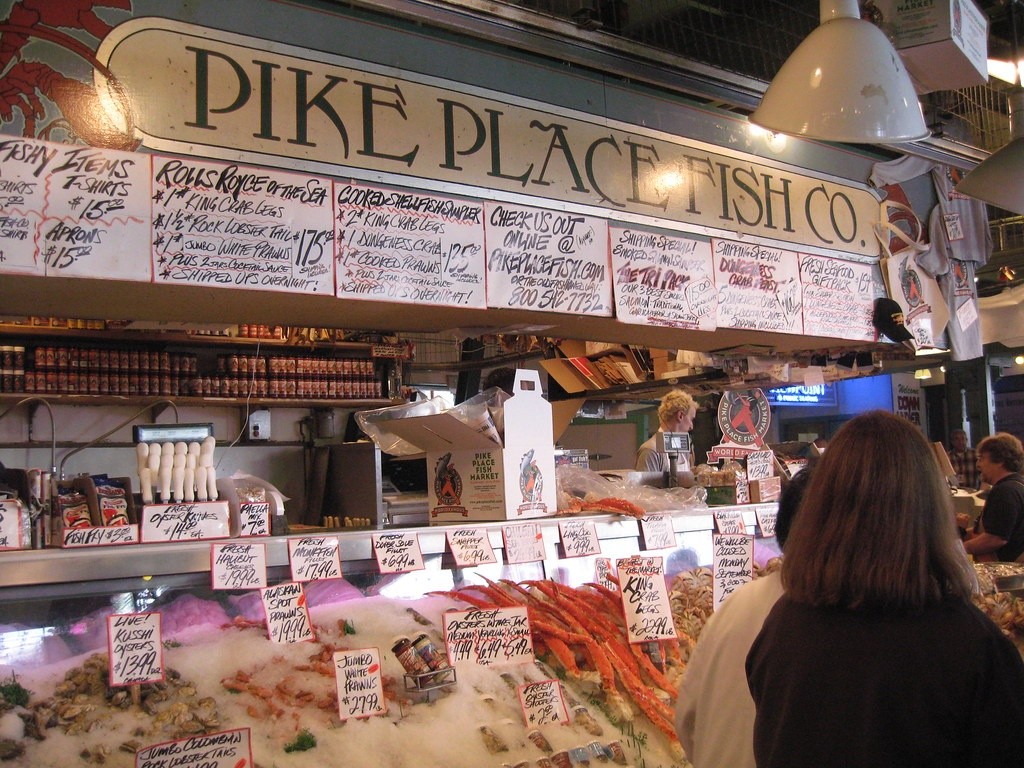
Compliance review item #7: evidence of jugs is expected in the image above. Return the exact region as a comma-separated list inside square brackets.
[299, 406, 334, 439]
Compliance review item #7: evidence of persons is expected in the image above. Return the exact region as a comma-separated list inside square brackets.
[949, 428, 987, 490]
[483, 366, 528, 408]
[675, 467, 812, 768]
[635, 390, 699, 487]
[961, 432, 1024, 562]
[745, 410, 1024, 768]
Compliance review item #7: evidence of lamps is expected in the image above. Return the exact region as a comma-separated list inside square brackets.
[61, 400, 213, 481]
[954, 135, 1024, 218]
[749, 0, 931, 143]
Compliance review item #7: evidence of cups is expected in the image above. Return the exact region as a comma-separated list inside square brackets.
[871, 351, 883, 374]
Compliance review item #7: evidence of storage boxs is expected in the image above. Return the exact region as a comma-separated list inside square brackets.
[762, 441, 820, 487]
[375, 367, 557, 524]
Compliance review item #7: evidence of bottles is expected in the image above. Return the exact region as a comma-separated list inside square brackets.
[1, 314, 381, 399]
[722, 458, 734, 470]
[392, 635, 451, 687]
[386, 370, 402, 400]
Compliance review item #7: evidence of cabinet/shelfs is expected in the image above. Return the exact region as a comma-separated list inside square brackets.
[0, 323, 401, 451]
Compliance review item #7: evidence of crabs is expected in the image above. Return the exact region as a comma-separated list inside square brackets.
[662, 557, 784, 645]
[424, 571, 687, 744]
